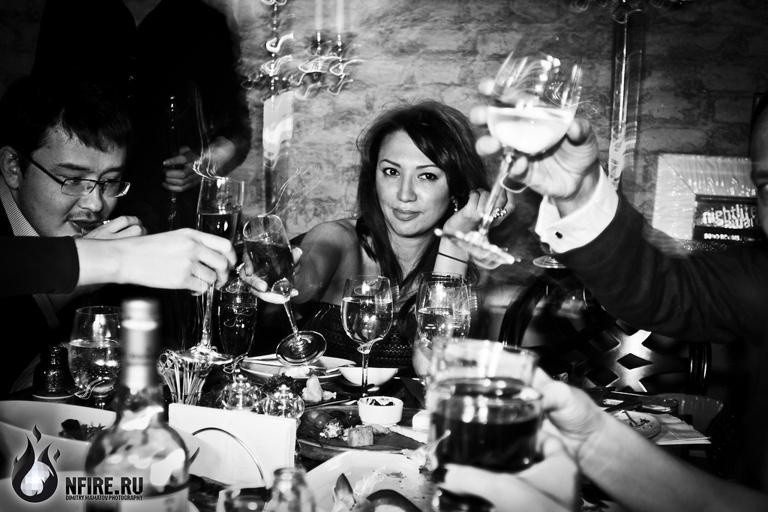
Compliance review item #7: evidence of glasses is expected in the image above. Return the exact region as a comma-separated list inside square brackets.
[26, 151, 131, 199]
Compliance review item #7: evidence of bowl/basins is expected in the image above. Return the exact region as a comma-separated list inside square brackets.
[411, 335, 517, 386]
[358, 395, 404, 426]
[0, 399, 119, 476]
[646, 393, 722, 434]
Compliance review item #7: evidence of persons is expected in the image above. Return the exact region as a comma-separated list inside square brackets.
[238, 97, 520, 384]
[0, 223, 242, 302]
[0, 85, 188, 401]
[463, 72, 768, 497]
[0, 0, 253, 339]
[401, 331, 767, 510]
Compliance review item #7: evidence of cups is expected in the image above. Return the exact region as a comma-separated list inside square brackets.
[420, 273, 542, 512]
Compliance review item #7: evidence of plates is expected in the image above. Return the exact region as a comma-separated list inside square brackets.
[241, 352, 357, 382]
[295, 405, 428, 462]
[341, 366, 399, 387]
[598, 406, 660, 440]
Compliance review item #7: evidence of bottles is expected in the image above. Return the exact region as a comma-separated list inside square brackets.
[85, 300, 188, 512]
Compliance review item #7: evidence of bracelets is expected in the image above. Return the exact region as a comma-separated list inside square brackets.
[435, 250, 468, 265]
[428, 272, 466, 290]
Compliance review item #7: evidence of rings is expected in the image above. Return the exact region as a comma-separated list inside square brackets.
[492, 207, 506, 219]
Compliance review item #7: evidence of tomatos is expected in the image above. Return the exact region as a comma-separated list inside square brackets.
[315, 415, 327, 428]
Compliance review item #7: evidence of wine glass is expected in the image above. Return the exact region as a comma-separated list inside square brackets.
[337, 273, 395, 402]
[181, 177, 327, 410]
[434, 45, 580, 267]
[67, 307, 126, 414]
